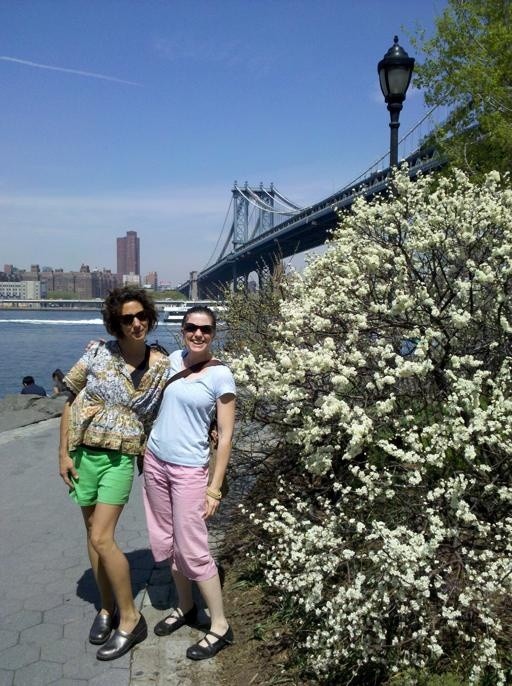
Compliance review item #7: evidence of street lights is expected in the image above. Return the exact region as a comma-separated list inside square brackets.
[377, 34, 416, 203]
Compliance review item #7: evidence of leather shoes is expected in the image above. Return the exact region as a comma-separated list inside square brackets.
[96, 611, 148, 660]
[185, 624, 233, 660]
[89, 605, 117, 645]
[154, 604, 197, 636]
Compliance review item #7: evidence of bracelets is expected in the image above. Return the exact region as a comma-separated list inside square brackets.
[207, 489, 222, 500]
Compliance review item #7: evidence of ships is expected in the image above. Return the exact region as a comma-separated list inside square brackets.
[162, 295, 231, 323]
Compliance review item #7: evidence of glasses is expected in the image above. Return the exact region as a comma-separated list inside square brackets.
[183, 323, 215, 334]
[121, 311, 148, 326]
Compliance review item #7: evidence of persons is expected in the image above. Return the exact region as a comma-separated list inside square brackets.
[21, 376, 46, 397]
[50, 368, 69, 396]
[59, 286, 167, 661]
[139, 305, 236, 660]
